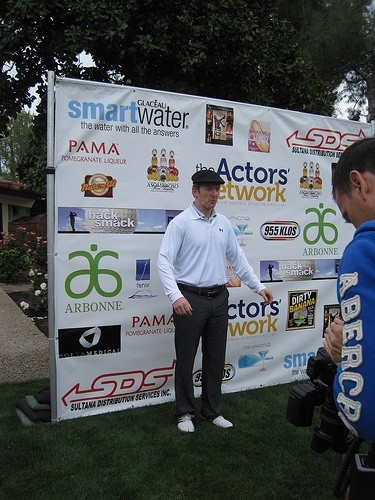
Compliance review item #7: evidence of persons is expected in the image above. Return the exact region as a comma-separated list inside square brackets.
[322, 134, 375, 500]
[158, 169, 274, 432]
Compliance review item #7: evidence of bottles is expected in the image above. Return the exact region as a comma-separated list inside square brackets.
[147, 149, 159, 175]
[168, 150, 179, 176]
[299, 162, 308, 183]
[314, 163, 322, 184]
[158, 148, 169, 175]
[308, 162, 315, 183]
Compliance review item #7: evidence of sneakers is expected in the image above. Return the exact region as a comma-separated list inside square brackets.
[209, 415, 233, 428]
[178, 413, 195, 432]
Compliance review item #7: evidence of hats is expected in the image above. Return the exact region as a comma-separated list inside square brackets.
[192, 170, 225, 186]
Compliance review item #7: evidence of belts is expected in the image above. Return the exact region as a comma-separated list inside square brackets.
[178, 284, 225, 299]
